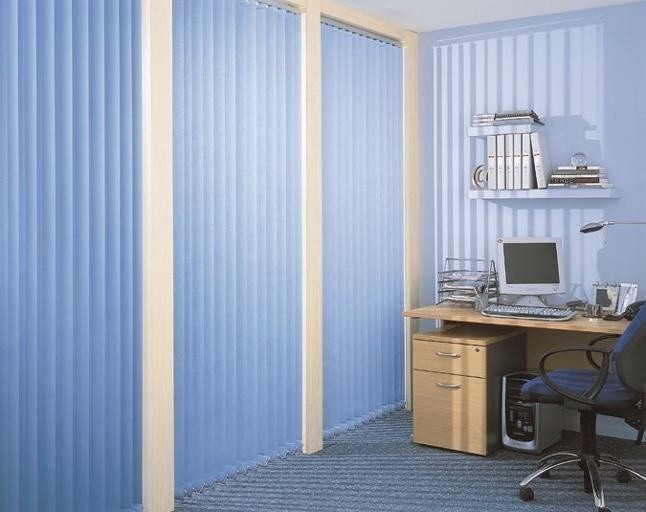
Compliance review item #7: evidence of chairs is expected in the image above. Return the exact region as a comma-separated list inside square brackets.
[520, 306, 645, 511]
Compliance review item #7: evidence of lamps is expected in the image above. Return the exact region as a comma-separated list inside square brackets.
[580, 219, 645, 233]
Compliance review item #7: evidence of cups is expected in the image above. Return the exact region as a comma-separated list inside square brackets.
[475, 294, 489, 311]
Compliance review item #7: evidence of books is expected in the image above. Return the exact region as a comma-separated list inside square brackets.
[486, 132, 613, 189]
[444, 269, 496, 305]
[472, 110, 547, 126]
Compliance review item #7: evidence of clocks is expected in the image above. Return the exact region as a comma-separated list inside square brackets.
[570, 153, 586, 167]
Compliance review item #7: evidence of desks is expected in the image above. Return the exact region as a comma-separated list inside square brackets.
[402, 294, 635, 457]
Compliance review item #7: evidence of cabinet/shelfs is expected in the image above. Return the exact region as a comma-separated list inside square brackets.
[468, 125, 619, 198]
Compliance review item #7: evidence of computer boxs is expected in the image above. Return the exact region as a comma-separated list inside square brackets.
[502, 371, 562, 454]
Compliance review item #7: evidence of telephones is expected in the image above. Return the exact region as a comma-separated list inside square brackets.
[626, 301, 646, 321]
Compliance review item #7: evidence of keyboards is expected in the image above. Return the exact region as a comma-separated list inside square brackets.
[480, 303, 576, 321]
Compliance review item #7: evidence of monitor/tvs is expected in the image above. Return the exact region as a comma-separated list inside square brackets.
[496, 237, 567, 308]
